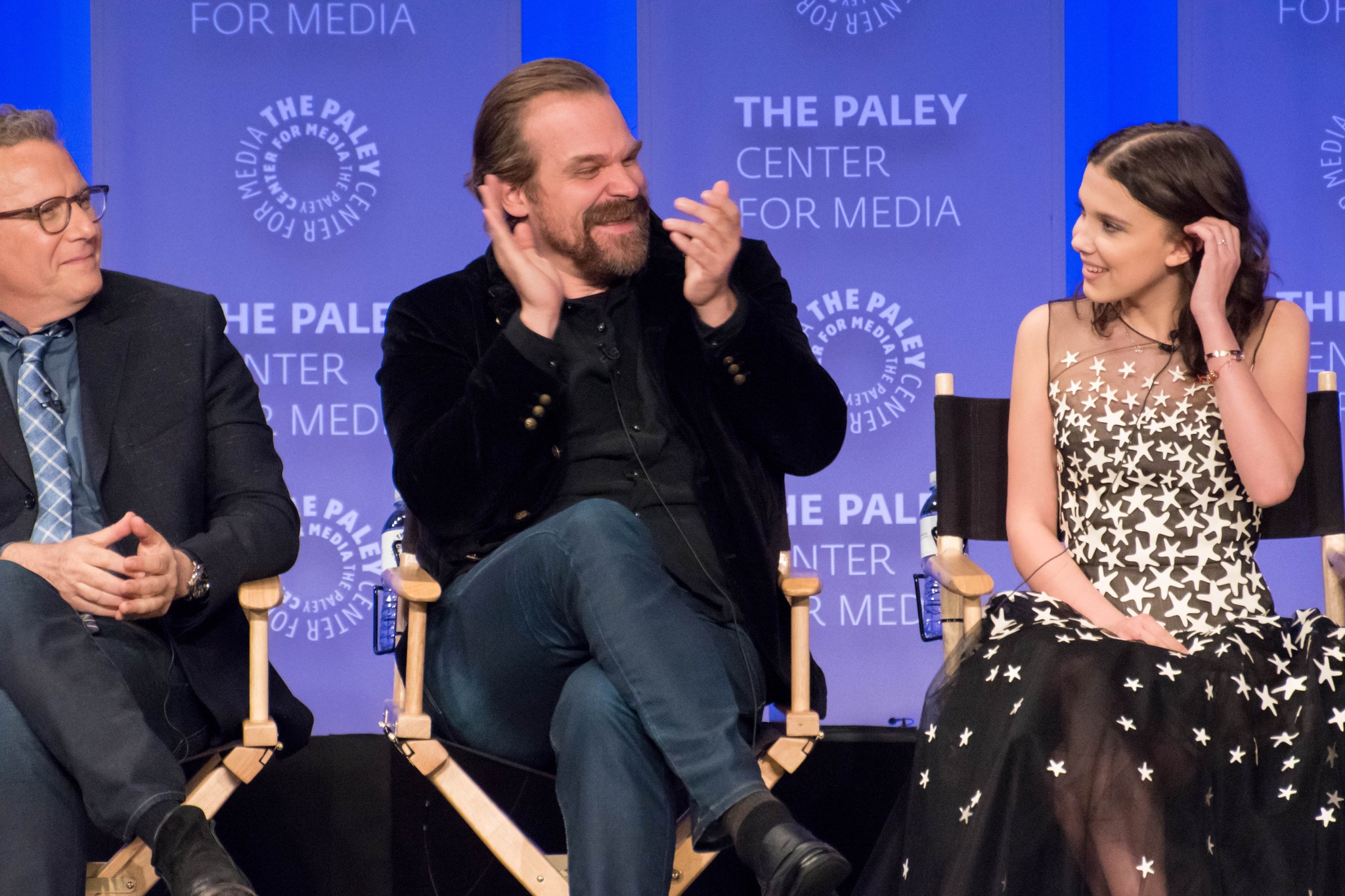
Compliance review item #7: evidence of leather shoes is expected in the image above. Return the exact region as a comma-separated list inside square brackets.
[754, 823, 851, 896]
[152, 804, 254, 896]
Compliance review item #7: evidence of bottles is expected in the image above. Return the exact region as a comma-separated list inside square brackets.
[379, 489, 408, 652]
[918, 471, 942, 636]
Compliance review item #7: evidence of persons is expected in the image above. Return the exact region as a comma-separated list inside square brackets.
[0, 103, 301, 896]
[373, 55, 855, 896]
[887, 123, 1344, 895]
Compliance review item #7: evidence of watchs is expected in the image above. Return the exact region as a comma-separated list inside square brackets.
[178, 545, 211, 601]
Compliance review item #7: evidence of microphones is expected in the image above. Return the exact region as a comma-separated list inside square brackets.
[41, 390, 64, 413]
[598, 343, 622, 359]
[1171, 330, 1184, 342]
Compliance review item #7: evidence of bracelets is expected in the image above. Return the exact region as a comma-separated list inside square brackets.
[1205, 354, 1246, 385]
[1204, 350, 1243, 358]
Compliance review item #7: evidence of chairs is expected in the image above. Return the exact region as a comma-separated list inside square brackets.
[86, 575, 285, 896]
[381, 503, 825, 896]
[924, 371, 1345, 677]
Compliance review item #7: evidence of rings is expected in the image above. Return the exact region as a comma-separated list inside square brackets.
[1217, 240, 1228, 247]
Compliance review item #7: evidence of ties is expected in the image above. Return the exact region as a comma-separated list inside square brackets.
[0, 320, 71, 543]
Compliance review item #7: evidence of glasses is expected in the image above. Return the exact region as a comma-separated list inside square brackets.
[0, 184, 110, 234]
